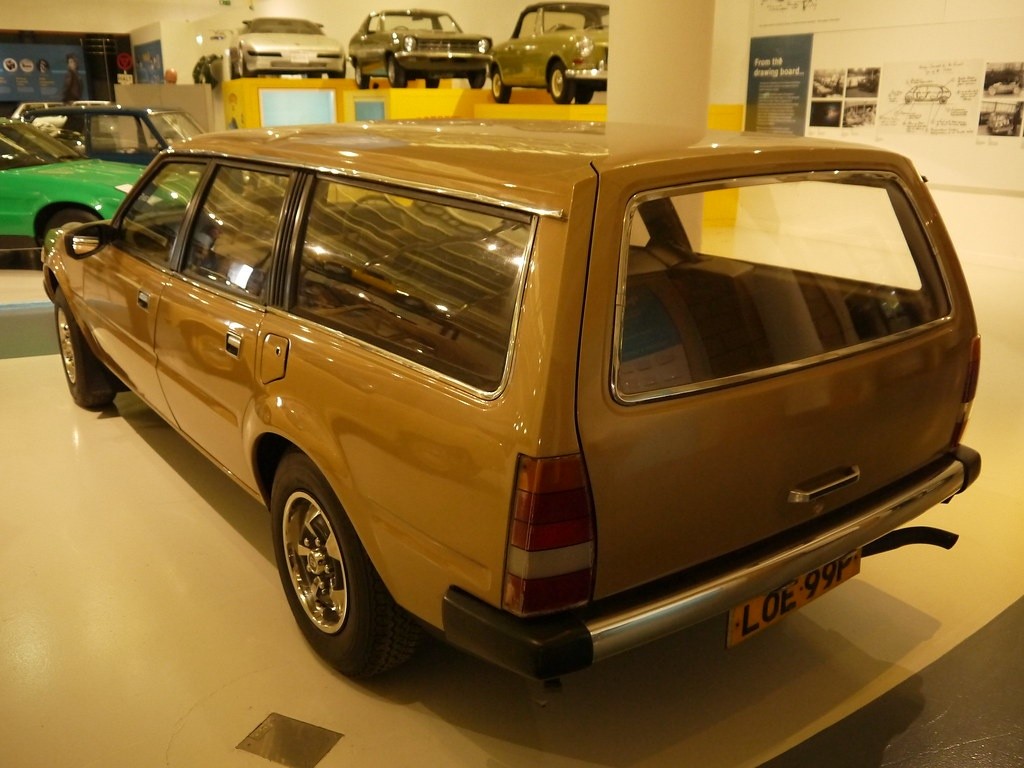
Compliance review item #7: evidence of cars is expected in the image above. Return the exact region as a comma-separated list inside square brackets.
[237, 16, 349, 79]
[43, 115, 983, 678]
[490, 5, 610, 104]
[349, 9, 494, 91]
[17, 106, 216, 172]
[1, 114, 200, 259]
